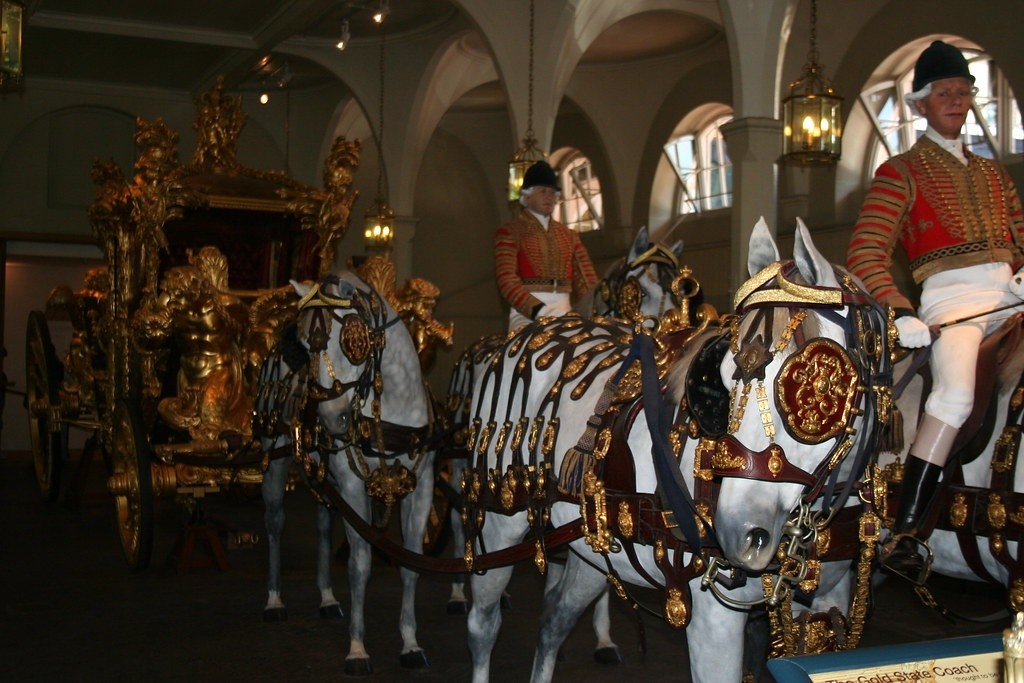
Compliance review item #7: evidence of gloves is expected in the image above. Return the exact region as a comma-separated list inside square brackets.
[535, 305, 565, 320]
[894, 316, 932, 349]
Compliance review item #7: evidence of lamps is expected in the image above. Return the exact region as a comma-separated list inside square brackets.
[507, 0, 547, 204]
[363, 8, 394, 254]
[336, 20, 351, 51]
[277, 72, 287, 87]
[0, 0, 23, 88]
[779, 0, 843, 166]
[370, 0, 391, 23]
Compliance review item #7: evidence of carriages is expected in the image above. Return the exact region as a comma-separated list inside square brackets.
[21, 75, 458, 679]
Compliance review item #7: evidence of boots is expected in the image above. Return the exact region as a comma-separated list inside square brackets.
[882, 453, 944, 576]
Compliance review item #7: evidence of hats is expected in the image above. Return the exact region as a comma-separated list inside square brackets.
[521, 160, 562, 190]
[912, 40, 975, 92]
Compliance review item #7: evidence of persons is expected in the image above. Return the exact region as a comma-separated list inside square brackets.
[496, 160, 600, 335]
[846, 41, 1024, 569]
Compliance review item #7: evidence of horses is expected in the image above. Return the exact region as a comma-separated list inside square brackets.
[440, 217, 1024, 683]
[251, 273, 430, 675]
